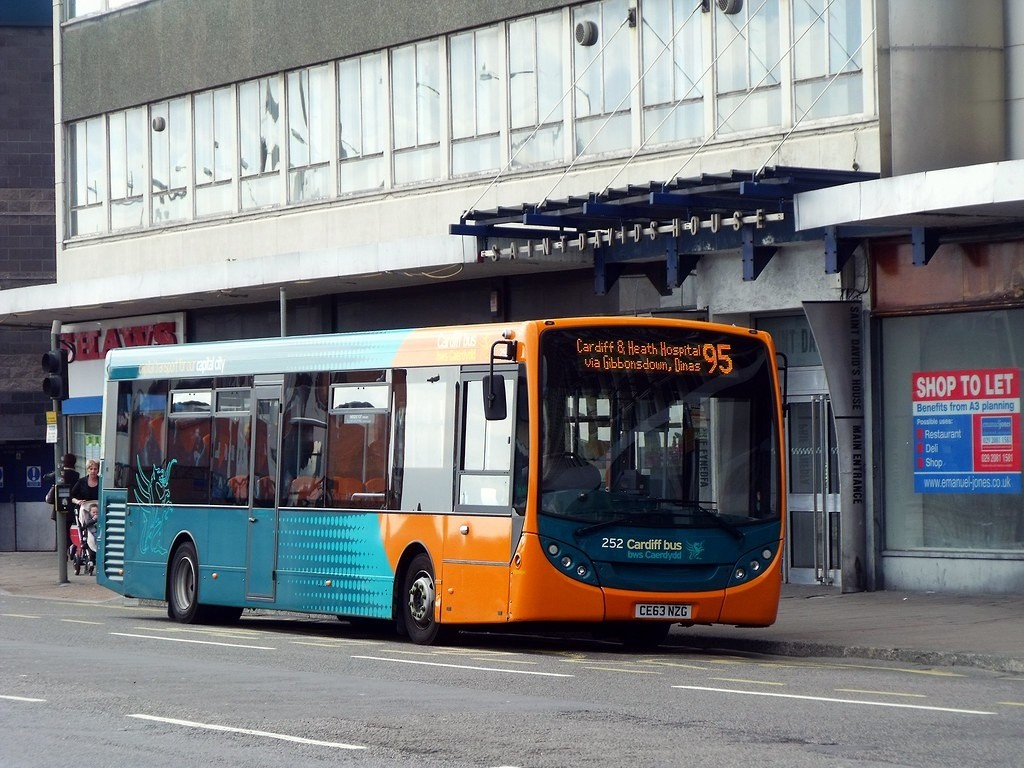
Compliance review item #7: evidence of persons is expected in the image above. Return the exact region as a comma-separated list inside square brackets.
[44, 454, 78, 562]
[70, 459, 100, 575]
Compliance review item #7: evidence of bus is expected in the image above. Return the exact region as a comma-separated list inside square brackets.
[91, 314, 795, 643]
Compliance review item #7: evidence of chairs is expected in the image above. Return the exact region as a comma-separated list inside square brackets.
[212, 470, 385, 508]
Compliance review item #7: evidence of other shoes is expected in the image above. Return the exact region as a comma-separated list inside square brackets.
[84, 570, 89, 574]
[69, 544, 77, 561]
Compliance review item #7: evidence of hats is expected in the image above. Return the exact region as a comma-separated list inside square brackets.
[63, 454, 77, 469]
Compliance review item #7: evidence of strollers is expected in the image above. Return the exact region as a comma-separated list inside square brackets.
[70, 498, 100, 576]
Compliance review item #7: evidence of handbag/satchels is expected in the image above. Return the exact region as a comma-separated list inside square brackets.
[45, 483, 55, 504]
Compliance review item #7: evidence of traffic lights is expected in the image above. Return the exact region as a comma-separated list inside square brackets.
[40, 347, 71, 401]
